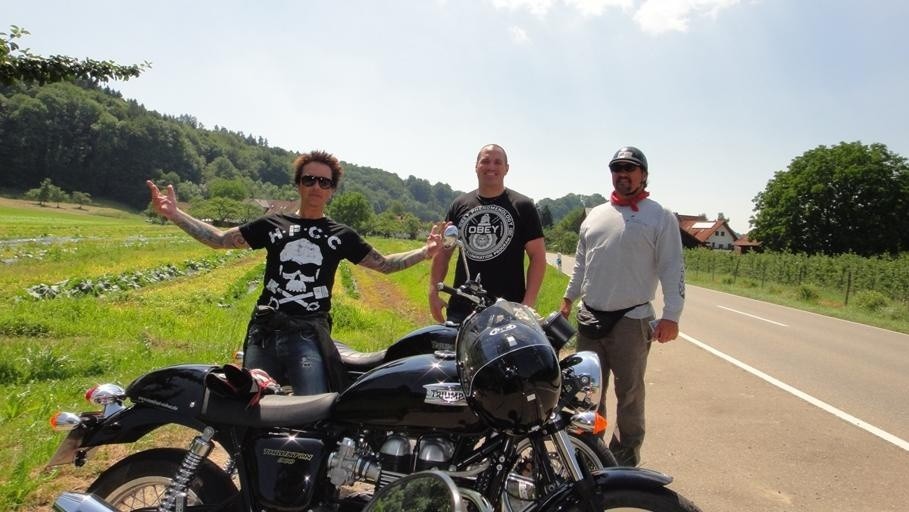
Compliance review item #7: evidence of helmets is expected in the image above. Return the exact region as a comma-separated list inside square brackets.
[609, 146, 648, 171]
[455, 301, 561, 436]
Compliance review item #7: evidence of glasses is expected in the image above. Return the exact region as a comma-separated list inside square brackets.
[611, 163, 637, 173]
[300, 174, 333, 190]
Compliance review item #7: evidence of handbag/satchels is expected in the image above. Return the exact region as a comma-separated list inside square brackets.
[574, 303, 634, 339]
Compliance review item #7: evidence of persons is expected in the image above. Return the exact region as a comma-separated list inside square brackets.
[427, 142, 549, 330]
[143, 149, 455, 398]
[559, 146, 688, 469]
